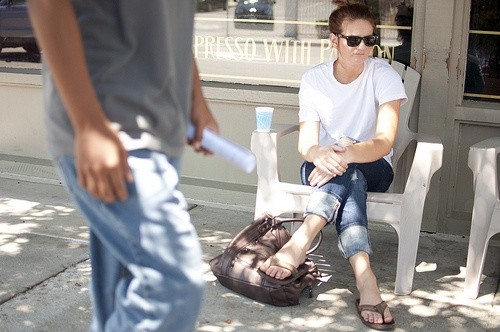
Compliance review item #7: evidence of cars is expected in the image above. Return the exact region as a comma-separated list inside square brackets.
[234, 0, 276, 31]
[0, 0, 41, 62]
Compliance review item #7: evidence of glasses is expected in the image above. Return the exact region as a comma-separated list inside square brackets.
[335, 33, 379, 47]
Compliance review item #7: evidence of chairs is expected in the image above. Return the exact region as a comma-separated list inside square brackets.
[460, 136, 500, 301]
[250, 57, 444, 296]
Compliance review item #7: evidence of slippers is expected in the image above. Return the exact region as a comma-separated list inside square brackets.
[356, 298, 396, 329]
[257, 254, 309, 284]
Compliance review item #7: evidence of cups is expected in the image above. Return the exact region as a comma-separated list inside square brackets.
[256, 107, 274, 133]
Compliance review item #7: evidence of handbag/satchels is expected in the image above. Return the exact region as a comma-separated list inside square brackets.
[209, 211, 323, 306]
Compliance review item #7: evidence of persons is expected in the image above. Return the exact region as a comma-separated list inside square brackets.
[26, 0, 218, 332]
[257, 0, 408, 332]
[382, 2, 414, 69]
